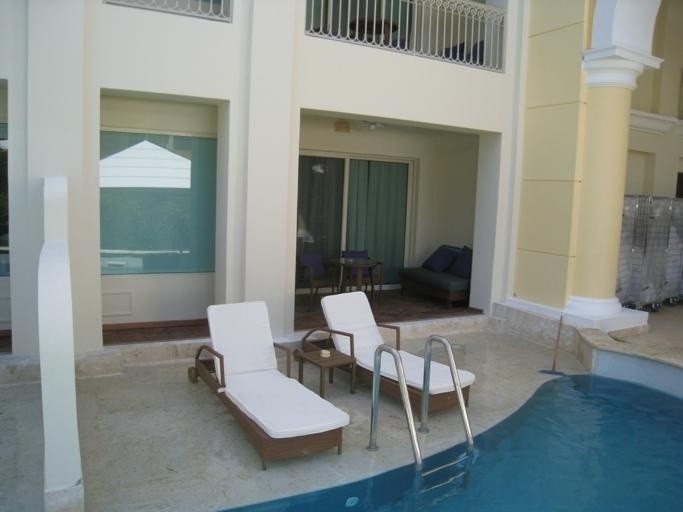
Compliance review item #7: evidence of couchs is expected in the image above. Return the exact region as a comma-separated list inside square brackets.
[399, 245, 473, 310]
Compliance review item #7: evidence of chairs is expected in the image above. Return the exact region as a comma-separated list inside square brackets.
[342, 250, 384, 292]
[321, 290, 476, 422]
[188, 299, 349, 470]
[303, 245, 338, 312]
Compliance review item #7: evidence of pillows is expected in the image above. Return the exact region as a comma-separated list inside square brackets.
[308, 246, 326, 279]
[451, 245, 472, 279]
[423, 246, 459, 273]
[346, 251, 369, 276]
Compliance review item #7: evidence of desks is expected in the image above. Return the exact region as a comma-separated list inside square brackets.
[298, 348, 357, 397]
[330, 257, 376, 302]
[350, 18, 398, 47]
